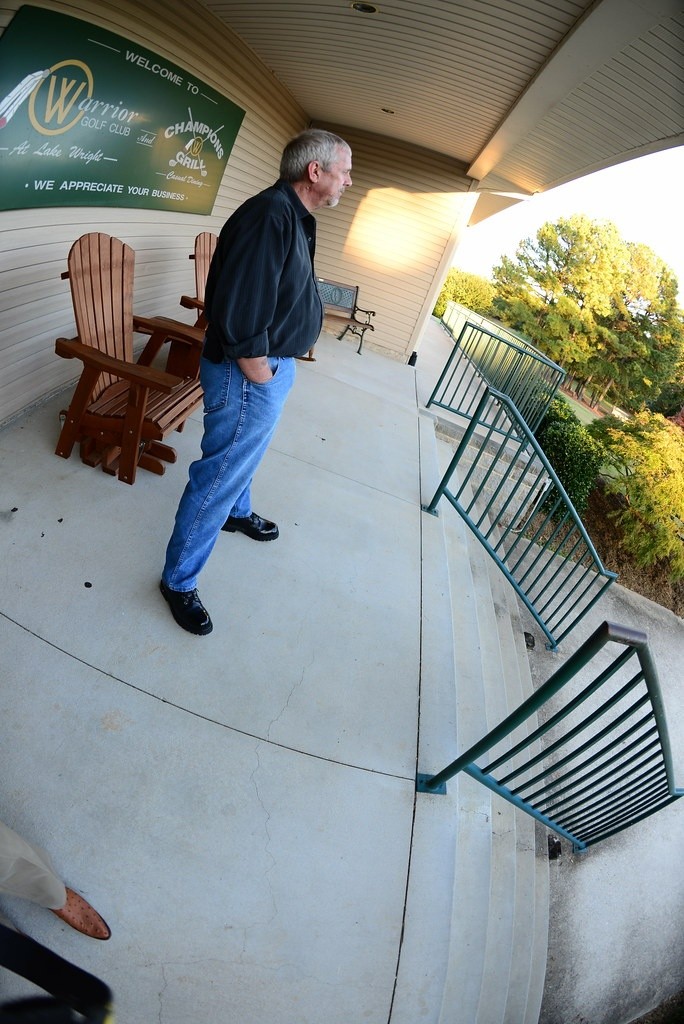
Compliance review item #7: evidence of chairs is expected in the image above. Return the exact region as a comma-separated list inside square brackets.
[180, 232, 317, 362]
[48, 232, 206, 486]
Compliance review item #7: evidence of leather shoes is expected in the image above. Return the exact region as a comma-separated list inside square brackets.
[46, 885, 111, 940]
[219, 511, 279, 542]
[158, 577, 214, 636]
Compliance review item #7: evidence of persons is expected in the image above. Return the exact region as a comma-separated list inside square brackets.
[158, 129, 354, 638]
[0, 820, 113, 941]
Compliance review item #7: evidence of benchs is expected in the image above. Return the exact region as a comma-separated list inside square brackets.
[315, 276, 376, 356]
